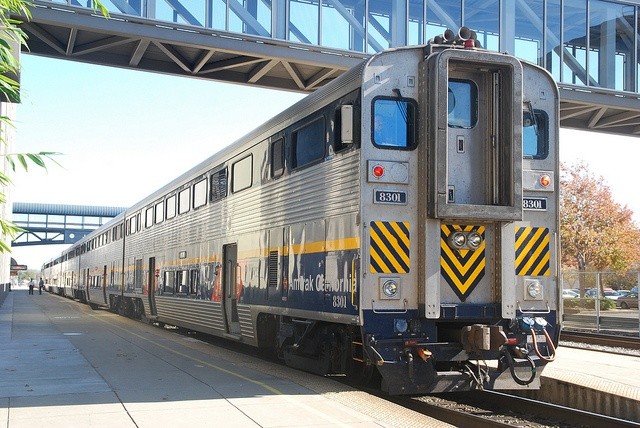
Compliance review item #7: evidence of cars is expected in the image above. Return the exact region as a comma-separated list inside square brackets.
[563, 283, 640, 309]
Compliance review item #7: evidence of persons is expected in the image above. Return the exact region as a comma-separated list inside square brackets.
[38, 277, 44, 295]
[374, 114, 385, 145]
[27, 277, 34, 294]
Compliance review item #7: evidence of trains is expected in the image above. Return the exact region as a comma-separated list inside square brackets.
[41, 25, 563, 403]
